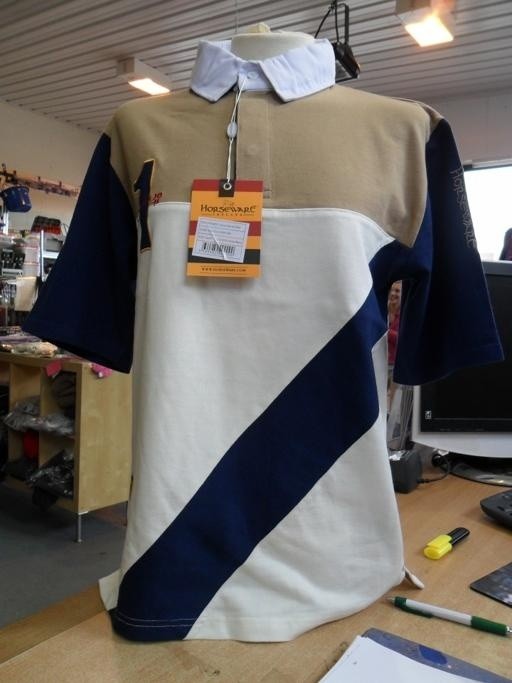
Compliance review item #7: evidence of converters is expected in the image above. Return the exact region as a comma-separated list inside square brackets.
[387, 449, 423, 493]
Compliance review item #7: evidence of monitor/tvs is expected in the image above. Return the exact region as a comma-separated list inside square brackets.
[410, 259, 512, 487]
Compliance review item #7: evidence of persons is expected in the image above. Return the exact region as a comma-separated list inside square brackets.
[19, 28, 506, 646]
[387, 279, 402, 419]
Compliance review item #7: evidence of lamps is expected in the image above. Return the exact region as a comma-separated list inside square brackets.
[396, 0, 456, 49]
[116, 57, 171, 95]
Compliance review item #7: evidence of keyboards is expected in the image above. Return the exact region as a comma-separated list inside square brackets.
[479, 489, 512, 531]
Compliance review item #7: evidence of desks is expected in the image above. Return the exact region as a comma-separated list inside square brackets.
[1, 470, 511, 683]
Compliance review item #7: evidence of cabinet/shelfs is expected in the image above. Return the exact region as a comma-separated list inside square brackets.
[0, 349, 133, 544]
[0, 229, 66, 282]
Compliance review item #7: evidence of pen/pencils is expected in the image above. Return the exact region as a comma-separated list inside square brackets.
[385, 595, 512, 635]
